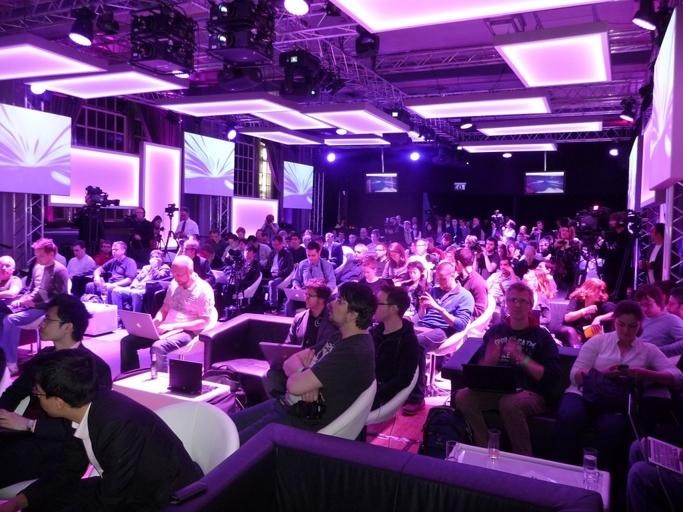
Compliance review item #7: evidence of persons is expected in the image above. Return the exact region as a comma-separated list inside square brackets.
[1, 207, 683, 511]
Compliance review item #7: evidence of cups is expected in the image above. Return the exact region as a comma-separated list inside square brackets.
[487, 430, 500, 460]
[446, 440, 457, 462]
[583, 447, 598, 480]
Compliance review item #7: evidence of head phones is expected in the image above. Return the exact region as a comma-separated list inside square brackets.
[617, 217, 626, 225]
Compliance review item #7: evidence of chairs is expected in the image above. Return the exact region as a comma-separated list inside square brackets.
[0, 245, 672, 512]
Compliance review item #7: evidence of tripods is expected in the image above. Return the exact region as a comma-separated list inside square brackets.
[219, 272, 246, 316]
[164, 231, 179, 252]
[614, 236, 651, 301]
[565, 244, 601, 300]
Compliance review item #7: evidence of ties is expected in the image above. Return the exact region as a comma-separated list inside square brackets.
[181, 222, 185, 231]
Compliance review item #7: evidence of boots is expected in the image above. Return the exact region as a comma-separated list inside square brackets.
[219, 308, 231, 321]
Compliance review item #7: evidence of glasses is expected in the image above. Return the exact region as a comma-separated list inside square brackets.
[42, 317, 62, 322]
[510, 296, 529, 306]
[304, 292, 319, 297]
[31, 387, 47, 397]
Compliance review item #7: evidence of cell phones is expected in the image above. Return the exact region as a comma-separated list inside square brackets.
[168, 482, 208, 505]
[618, 364, 628, 371]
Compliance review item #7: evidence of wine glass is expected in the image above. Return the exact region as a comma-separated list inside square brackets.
[101, 285, 108, 307]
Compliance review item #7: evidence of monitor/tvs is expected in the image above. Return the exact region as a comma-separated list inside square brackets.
[363, 171, 399, 193]
[523, 171, 568, 196]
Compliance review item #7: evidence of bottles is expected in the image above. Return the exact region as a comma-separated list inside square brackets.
[150, 353, 157, 379]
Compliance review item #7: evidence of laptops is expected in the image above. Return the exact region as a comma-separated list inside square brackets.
[119, 310, 166, 340]
[168, 359, 218, 397]
[628, 393, 683, 475]
[461, 364, 524, 394]
[284, 288, 307, 301]
[259, 342, 304, 370]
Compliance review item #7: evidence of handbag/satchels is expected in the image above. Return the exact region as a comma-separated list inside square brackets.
[462, 362, 517, 394]
[582, 367, 636, 411]
[285, 390, 326, 420]
[267, 365, 286, 397]
[417, 405, 466, 458]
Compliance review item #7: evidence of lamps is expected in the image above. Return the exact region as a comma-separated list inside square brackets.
[619, 100, 637, 122]
[68, 7, 93, 47]
[459, 117, 473, 130]
[225, 122, 238, 140]
[631, 0, 657, 31]
